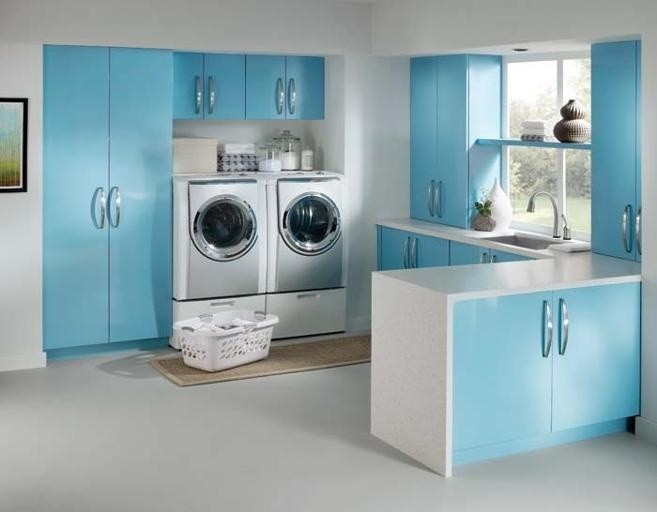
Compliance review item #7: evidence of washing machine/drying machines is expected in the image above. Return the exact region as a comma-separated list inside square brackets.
[172, 175, 267, 301]
[267, 170, 351, 294]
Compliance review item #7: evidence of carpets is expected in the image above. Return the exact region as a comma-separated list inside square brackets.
[145, 332, 370, 387]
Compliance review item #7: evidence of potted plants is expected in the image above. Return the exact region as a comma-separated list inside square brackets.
[472, 200, 496, 232]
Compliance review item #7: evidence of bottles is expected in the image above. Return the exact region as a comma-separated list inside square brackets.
[258, 143, 282, 172]
[301, 145, 314, 171]
[273, 129, 302, 171]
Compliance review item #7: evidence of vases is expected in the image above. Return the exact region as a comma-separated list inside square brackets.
[487, 177, 513, 230]
[554, 97, 591, 142]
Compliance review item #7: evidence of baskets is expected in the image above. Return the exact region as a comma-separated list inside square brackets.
[173, 309, 280, 373]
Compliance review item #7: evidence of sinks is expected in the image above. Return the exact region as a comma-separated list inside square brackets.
[482, 236, 565, 251]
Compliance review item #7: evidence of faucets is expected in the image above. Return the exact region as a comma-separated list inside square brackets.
[526, 191, 561, 237]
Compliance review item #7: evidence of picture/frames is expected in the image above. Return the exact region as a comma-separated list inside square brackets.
[0, 96, 29, 193]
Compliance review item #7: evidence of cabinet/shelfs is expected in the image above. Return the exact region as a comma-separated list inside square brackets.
[450, 239, 537, 266]
[452, 279, 640, 467]
[409, 53, 501, 229]
[42, 44, 173, 350]
[175, 50, 244, 121]
[590, 40, 641, 262]
[376, 225, 449, 271]
[243, 53, 325, 120]
